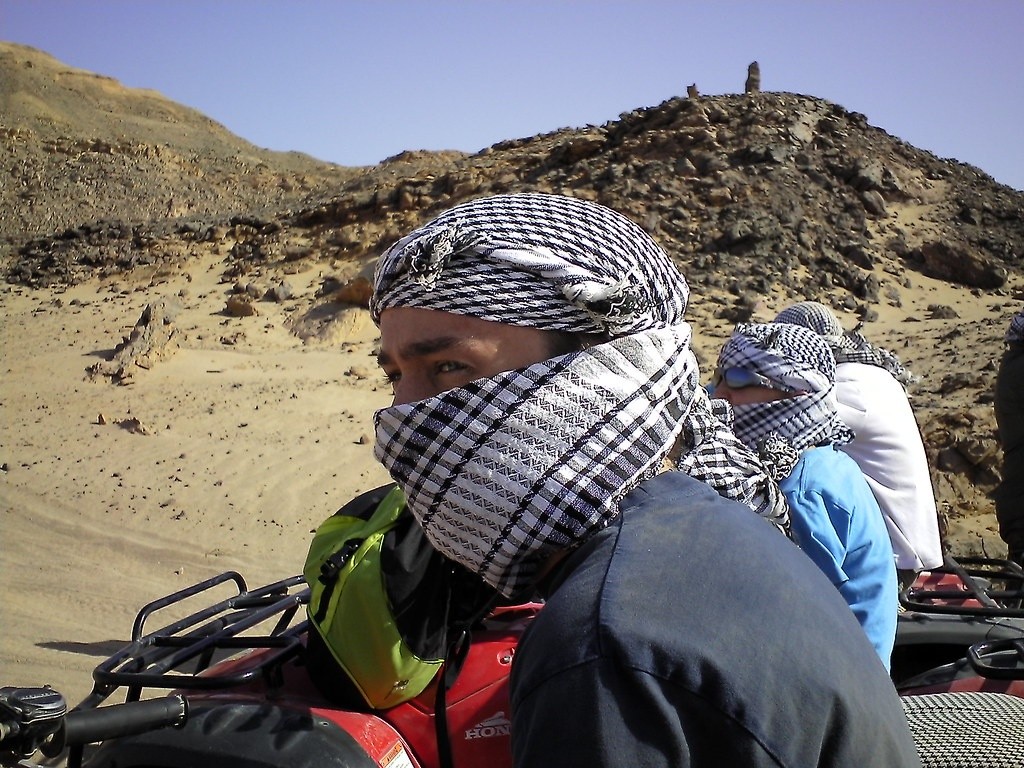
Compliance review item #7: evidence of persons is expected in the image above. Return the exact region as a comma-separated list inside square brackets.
[993, 309, 1024, 609]
[774, 301, 943, 589]
[710, 322, 898, 678]
[369, 193, 924, 768]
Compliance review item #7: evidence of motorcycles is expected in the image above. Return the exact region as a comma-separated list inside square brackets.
[1, 551, 1024, 768]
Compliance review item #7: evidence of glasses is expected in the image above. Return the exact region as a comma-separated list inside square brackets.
[713, 367, 790, 393]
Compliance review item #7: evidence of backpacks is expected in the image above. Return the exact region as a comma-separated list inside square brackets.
[302, 480, 496, 768]
